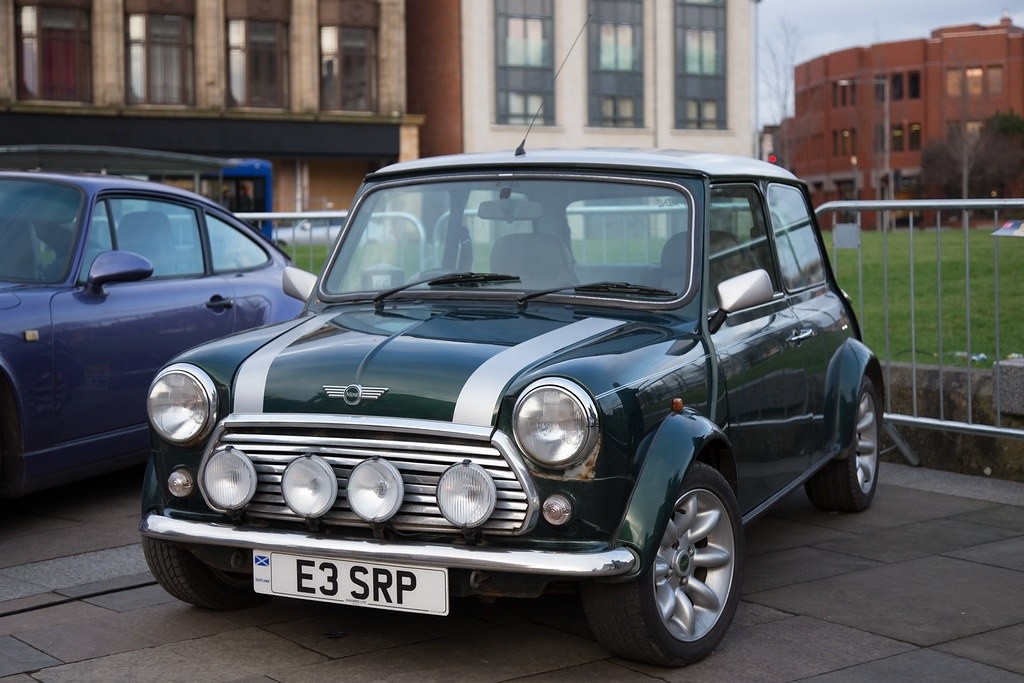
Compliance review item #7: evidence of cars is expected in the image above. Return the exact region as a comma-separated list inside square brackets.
[0, 169, 309, 498]
[134, 142, 887, 670]
[272, 217, 385, 250]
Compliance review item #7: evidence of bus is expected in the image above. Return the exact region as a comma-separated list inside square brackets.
[0, 143, 276, 246]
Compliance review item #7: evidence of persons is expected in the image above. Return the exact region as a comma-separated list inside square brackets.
[31, 188, 106, 285]
[219, 182, 250, 213]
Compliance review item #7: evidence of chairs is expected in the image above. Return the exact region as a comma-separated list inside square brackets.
[661, 232, 759, 306]
[117, 211, 177, 275]
[489, 233, 574, 292]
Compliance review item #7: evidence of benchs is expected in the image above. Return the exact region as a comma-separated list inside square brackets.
[575, 264, 660, 287]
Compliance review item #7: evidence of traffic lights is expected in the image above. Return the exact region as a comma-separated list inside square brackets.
[768, 152, 777, 165]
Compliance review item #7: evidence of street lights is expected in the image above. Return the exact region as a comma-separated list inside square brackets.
[838, 75, 891, 231]
